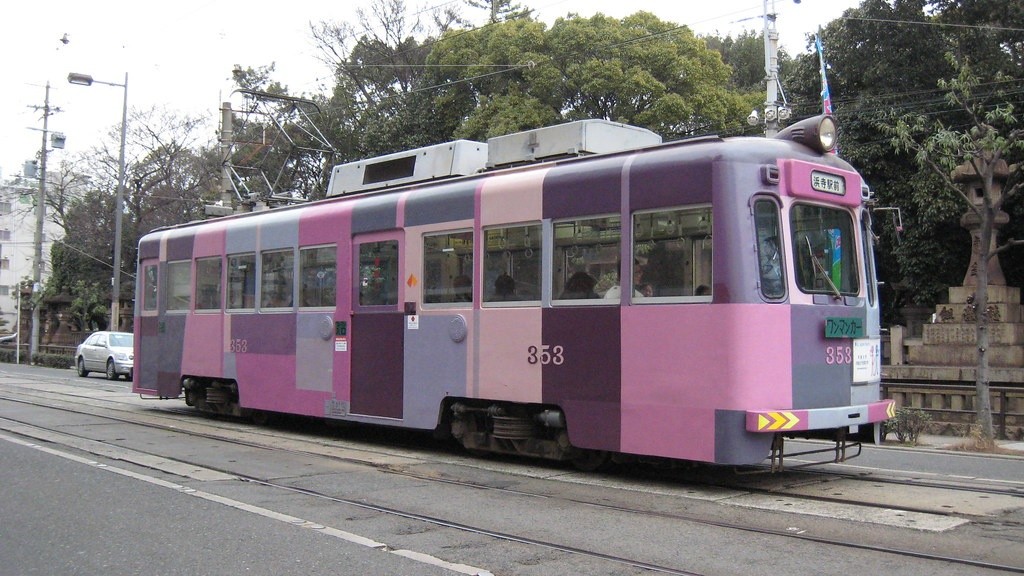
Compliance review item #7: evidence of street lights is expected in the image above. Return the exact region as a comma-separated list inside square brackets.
[67, 72, 129, 331]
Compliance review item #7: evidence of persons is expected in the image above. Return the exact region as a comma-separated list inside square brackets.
[195, 284, 218, 309]
[638, 282, 657, 297]
[289, 283, 310, 307]
[487, 276, 522, 302]
[695, 284, 712, 295]
[603, 258, 644, 299]
[446, 275, 472, 302]
[763, 236, 805, 287]
[559, 271, 600, 300]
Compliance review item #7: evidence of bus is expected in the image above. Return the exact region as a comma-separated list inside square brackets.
[130, 111, 904, 469]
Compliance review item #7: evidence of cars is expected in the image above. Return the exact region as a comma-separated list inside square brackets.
[74, 330, 134, 380]
[0, 332, 18, 344]
